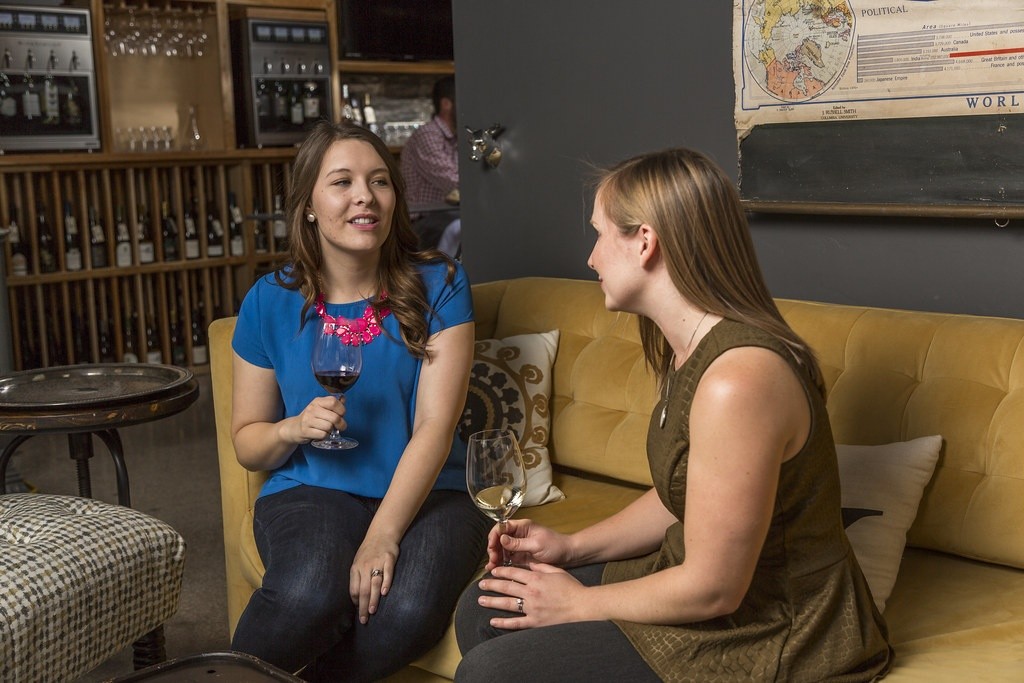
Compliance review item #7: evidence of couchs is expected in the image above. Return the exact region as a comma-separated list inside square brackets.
[208, 277, 1024, 683]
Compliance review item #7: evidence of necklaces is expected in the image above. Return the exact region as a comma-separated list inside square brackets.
[660, 311, 708, 428]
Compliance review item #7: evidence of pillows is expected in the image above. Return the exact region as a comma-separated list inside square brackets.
[836, 435, 943, 614]
[455, 328, 566, 510]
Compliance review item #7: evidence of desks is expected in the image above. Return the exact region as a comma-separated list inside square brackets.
[1, 363, 201, 508]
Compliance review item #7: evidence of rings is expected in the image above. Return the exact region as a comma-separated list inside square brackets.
[517, 598, 523, 613]
[371, 570, 383, 577]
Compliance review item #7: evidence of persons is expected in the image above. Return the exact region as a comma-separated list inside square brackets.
[454, 149, 897, 683]
[402, 76, 462, 256]
[230, 125, 489, 683]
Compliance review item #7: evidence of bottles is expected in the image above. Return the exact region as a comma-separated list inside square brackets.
[98, 313, 208, 369]
[112, 104, 203, 155]
[8, 195, 288, 277]
[340, 84, 378, 135]
[0, 71, 85, 134]
[256, 79, 320, 133]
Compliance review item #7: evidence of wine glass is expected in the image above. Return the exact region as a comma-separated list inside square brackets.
[464, 428, 529, 585]
[103, 3, 210, 60]
[310, 321, 363, 448]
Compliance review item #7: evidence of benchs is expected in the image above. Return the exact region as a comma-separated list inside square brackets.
[0, 491, 187, 683]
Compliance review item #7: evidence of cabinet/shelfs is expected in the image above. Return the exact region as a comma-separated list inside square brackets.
[0, 144, 413, 374]
